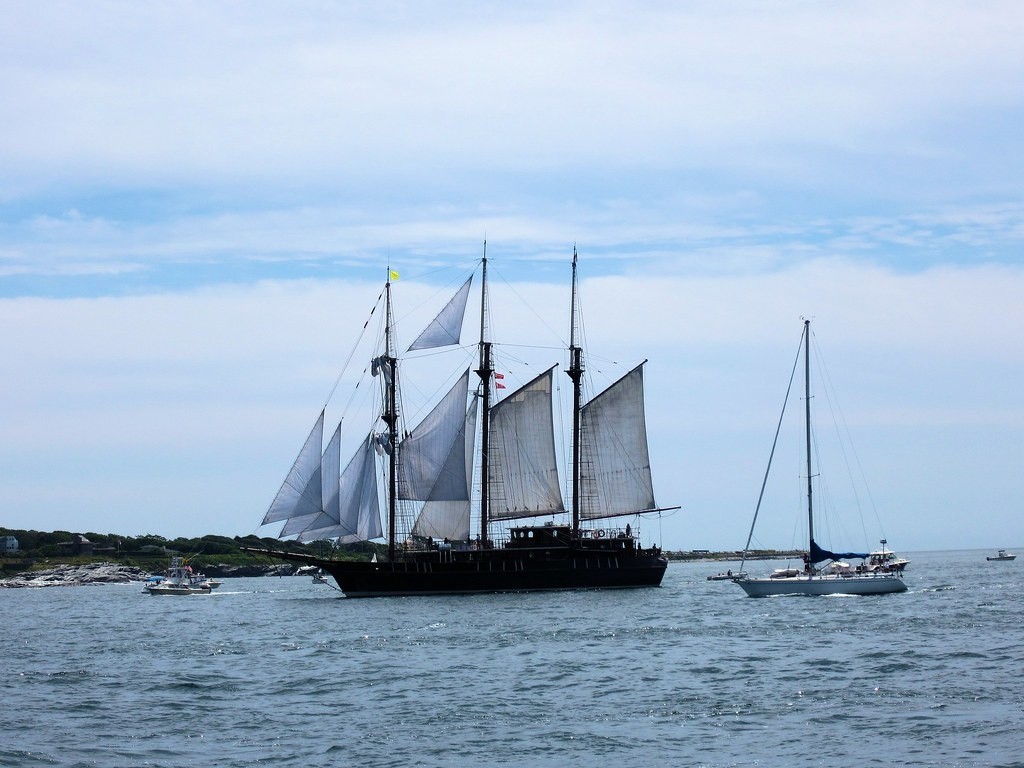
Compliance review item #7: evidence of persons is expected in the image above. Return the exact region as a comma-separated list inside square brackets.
[476, 534, 480, 550]
[626, 524, 630, 538]
[157, 580, 161, 585]
[728, 568, 732, 576]
[444, 537, 448, 544]
[467, 535, 470, 540]
[885, 558, 889, 577]
[426, 536, 432, 551]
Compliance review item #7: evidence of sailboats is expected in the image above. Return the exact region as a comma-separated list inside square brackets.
[730, 314, 908, 600]
[236, 227, 683, 599]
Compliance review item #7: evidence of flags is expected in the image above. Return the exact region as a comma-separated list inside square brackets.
[390, 270, 398, 280]
[495, 372, 504, 380]
[496, 382, 506, 388]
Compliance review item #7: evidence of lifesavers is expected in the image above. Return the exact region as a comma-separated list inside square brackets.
[593, 531, 599, 539]
[598, 530, 605, 537]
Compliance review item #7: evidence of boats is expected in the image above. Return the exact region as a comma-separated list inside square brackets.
[310, 573, 330, 585]
[855, 538, 910, 574]
[706, 557, 746, 581]
[987, 550, 1017, 562]
[140, 550, 225, 595]
[767, 565, 801, 577]
